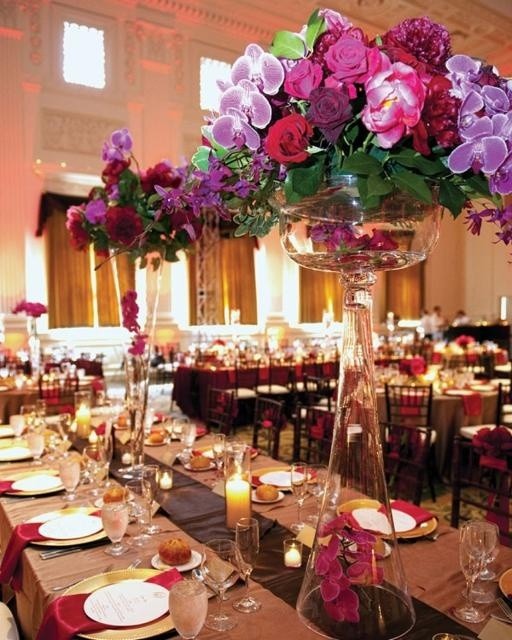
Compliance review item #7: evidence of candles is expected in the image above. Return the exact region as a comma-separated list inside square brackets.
[121, 452, 132, 464]
[90, 431, 98, 443]
[284, 544, 300, 565]
[160, 472, 172, 489]
[78, 407, 91, 438]
[225, 472, 251, 530]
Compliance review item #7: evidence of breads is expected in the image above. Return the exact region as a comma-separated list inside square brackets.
[103, 485, 129, 504]
[80, 456, 89, 471]
[191, 455, 210, 468]
[149, 433, 163, 444]
[118, 416, 127, 427]
[255, 484, 279, 501]
[374, 536, 385, 555]
[159, 537, 191, 565]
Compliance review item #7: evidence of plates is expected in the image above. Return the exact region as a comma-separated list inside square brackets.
[113, 424, 130, 429]
[0, 448, 32, 460]
[350, 540, 391, 558]
[151, 549, 202, 572]
[4, 470, 64, 496]
[95, 498, 104, 508]
[18, 507, 109, 545]
[153, 427, 206, 439]
[491, 379, 510, 386]
[446, 389, 473, 395]
[260, 472, 304, 486]
[248, 467, 318, 490]
[55, 569, 176, 640]
[11, 477, 61, 491]
[251, 491, 285, 503]
[185, 462, 215, 471]
[471, 385, 494, 391]
[0, 427, 14, 437]
[500, 568, 512, 602]
[38, 515, 103, 539]
[353, 508, 416, 532]
[337, 499, 437, 538]
[196, 444, 257, 460]
[144, 437, 167, 446]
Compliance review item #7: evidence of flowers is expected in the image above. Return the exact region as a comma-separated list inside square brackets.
[119, 289, 149, 456]
[313, 509, 387, 625]
[92, 6, 511, 269]
[10, 299, 47, 318]
[65, 128, 204, 268]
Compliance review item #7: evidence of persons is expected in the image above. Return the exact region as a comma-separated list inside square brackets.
[419, 307, 433, 340]
[453, 310, 471, 327]
[429, 306, 449, 340]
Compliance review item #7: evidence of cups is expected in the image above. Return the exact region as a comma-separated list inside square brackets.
[226, 445, 252, 530]
[283, 539, 303, 567]
[121, 447, 132, 465]
[84, 581, 170, 626]
[74, 391, 93, 440]
[432, 633, 460, 640]
[159, 470, 173, 490]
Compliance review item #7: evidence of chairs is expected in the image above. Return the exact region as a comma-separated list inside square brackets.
[378, 421, 429, 506]
[450, 434, 511, 548]
[225, 359, 259, 436]
[384, 383, 435, 503]
[302, 372, 330, 412]
[252, 396, 284, 459]
[252, 355, 292, 395]
[205, 388, 233, 435]
[288, 356, 323, 392]
[459, 383, 511, 439]
[292, 401, 335, 467]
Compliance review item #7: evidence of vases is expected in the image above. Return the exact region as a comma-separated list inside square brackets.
[275, 174, 441, 640]
[107, 240, 166, 477]
[29, 315, 42, 374]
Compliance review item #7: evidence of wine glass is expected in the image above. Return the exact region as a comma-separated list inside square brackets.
[96, 436, 113, 489]
[454, 525, 485, 622]
[213, 434, 226, 486]
[11, 415, 25, 441]
[290, 463, 309, 531]
[168, 580, 208, 640]
[235, 519, 259, 613]
[36, 400, 47, 431]
[58, 413, 71, 460]
[224, 436, 243, 480]
[462, 523, 496, 604]
[470, 522, 499, 581]
[142, 465, 159, 534]
[21, 405, 37, 439]
[174, 418, 188, 454]
[103, 502, 130, 556]
[124, 480, 152, 546]
[304, 464, 328, 523]
[61, 462, 80, 500]
[181, 424, 195, 463]
[28, 436, 45, 465]
[83, 445, 106, 497]
[201, 540, 241, 630]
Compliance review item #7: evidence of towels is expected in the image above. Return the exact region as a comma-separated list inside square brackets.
[191, 446, 256, 461]
[0, 511, 109, 591]
[400, 395, 420, 417]
[378, 499, 439, 526]
[196, 426, 208, 434]
[251, 466, 317, 488]
[35, 568, 184, 640]
[0, 480, 19, 497]
[445, 389, 481, 415]
